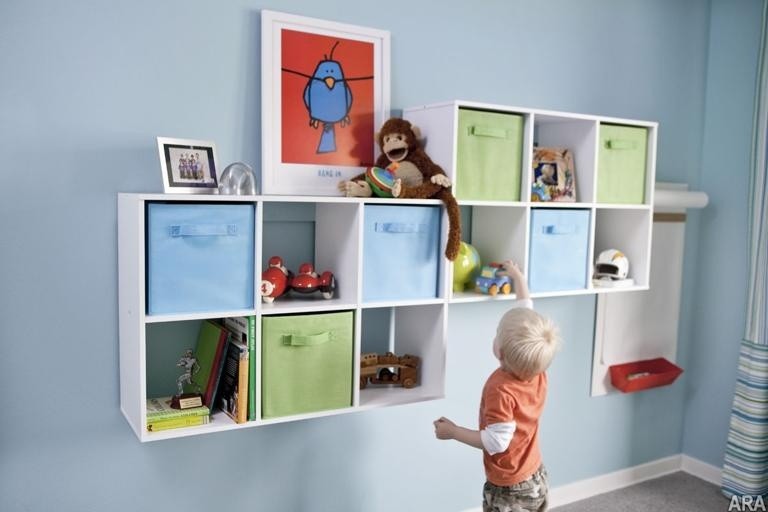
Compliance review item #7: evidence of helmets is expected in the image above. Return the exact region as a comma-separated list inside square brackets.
[594, 249, 629, 279]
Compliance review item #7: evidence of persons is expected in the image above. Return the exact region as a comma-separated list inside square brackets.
[178, 151, 206, 179]
[337, 117, 462, 259]
[535, 162, 558, 185]
[432, 259, 558, 512]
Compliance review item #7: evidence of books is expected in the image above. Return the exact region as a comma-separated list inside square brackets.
[145, 315, 257, 432]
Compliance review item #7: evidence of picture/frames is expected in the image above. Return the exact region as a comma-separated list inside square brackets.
[259, 8, 392, 198]
